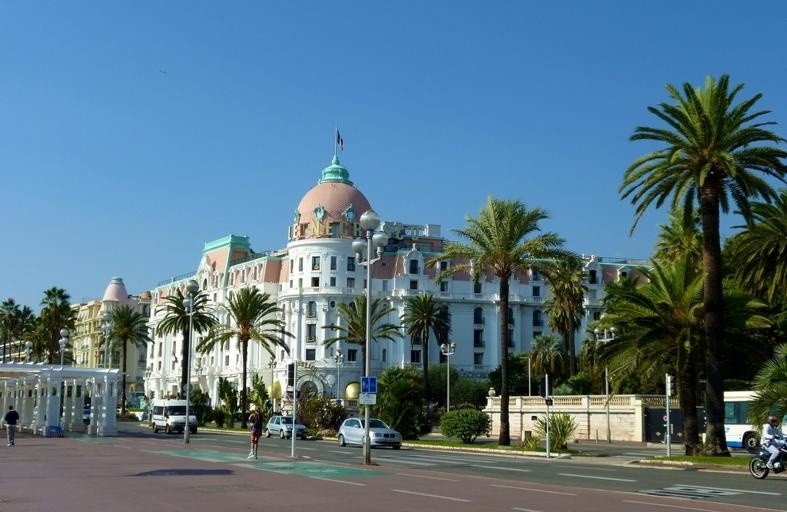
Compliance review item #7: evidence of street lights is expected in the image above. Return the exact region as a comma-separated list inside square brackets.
[593, 325, 618, 443]
[192, 357, 203, 383]
[144, 366, 151, 391]
[22, 341, 35, 363]
[333, 350, 344, 401]
[58, 324, 68, 365]
[267, 354, 277, 399]
[440, 341, 455, 412]
[99, 312, 114, 369]
[349, 207, 389, 464]
[182, 276, 202, 445]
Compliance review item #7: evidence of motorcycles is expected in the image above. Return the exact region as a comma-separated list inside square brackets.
[749, 436, 787, 481]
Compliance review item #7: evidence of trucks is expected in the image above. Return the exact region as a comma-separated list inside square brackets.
[150, 396, 199, 435]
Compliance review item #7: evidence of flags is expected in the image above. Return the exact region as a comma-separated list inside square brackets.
[337, 131, 343, 152]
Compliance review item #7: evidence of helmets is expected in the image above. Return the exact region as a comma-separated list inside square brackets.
[766, 416, 778, 427]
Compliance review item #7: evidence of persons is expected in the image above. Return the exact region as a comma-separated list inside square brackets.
[245, 405, 263, 460]
[2, 404, 20, 446]
[758, 415, 786, 470]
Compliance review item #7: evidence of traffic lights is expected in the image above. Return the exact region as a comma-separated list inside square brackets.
[667, 374, 677, 398]
[544, 398, 553, 406]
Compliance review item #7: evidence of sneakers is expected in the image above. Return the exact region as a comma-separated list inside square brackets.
[766, 462, 774, 470]
[248, 453, 255, 459]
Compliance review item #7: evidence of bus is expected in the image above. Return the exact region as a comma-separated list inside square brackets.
[700, 389, 787, 454]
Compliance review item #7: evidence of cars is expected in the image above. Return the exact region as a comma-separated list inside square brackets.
[264, 414, 306, 440]
[32, 403, 62, 420]
[336, 415, 403, 451]
[83, 408, 91, 422]
